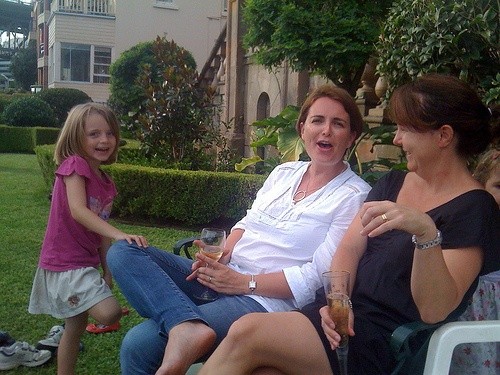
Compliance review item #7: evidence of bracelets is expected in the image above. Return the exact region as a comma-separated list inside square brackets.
[328, 293, 353, 308]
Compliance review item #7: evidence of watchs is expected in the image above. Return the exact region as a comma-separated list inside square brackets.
[411, 230, 442, 249]
[248, 274, 257, 294]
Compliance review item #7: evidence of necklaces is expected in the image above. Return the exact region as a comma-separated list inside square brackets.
[293, 166, 344, 202]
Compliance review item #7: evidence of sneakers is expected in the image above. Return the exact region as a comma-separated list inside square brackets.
[0, 340, 52, 370]
[37, 323, 84, 353]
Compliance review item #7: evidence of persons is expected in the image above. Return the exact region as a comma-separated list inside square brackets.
[198, 75, 500, 375]
[473, 148, 500, 209]
[106, 84, 372, 375]
[28, 104, 148, 375]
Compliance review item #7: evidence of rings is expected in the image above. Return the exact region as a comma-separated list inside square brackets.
[381, 214, 387, 222]
[209, 277, 212, 282]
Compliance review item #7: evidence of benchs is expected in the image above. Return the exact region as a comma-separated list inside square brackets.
[174, 233, 499, 375]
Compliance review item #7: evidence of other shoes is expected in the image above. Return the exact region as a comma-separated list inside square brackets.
[87, 322, 120, 333]
[1, 331, 15, 346]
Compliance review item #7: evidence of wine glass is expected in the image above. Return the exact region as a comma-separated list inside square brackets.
[192, 226, 228, 301]
[322, 269, 351, 375]
